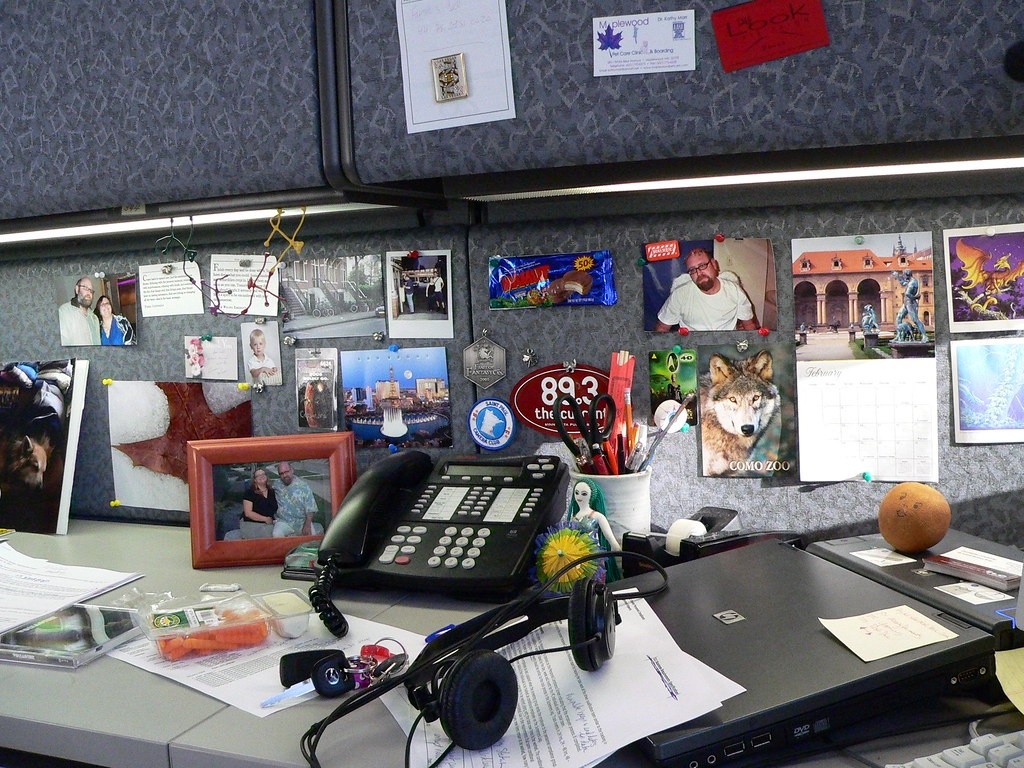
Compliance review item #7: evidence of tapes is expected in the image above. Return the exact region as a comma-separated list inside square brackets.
[665, 518, 707, 555]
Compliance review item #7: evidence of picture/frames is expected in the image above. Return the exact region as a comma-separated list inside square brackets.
[187, 431, 355, 569]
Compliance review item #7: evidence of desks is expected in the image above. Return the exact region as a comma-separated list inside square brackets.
[0, 518, 527, 768]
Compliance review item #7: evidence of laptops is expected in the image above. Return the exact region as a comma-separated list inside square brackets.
[807, 526, 1024, 651]
[530, 536, 995, 768]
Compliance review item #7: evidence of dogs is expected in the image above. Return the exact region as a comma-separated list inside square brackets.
[0, 431, 53, 491]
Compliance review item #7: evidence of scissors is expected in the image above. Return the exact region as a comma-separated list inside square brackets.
[553, 394, 618, 456]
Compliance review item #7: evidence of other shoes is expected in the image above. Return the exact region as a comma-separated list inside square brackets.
[443, 309, 447, 314]
[436, 307, 441, 312]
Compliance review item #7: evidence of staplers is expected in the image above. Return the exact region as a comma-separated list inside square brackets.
[679, 528, 808, 570]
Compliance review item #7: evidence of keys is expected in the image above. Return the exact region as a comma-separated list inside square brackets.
[258, 642, 411, 711]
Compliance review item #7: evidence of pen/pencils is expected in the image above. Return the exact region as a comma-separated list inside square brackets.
[574, 348, 694, 474]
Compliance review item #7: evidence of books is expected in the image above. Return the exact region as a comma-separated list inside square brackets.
[923, 546, 1024, 593]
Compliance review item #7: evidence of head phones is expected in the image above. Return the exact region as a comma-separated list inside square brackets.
[403, 576, 623, 750]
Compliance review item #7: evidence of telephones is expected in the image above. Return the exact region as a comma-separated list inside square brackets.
[317, 447, 568, 603]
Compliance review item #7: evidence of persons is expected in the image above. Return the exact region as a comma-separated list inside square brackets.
[800, 321, 864, 334]
[655, 248, 757, 332]
[248, 329, 281, 386]
[862, 268, 930, 344]
[238, 460, 319, 539]
[59, 277, 134, 345]
[561, 478, 621, 589]
[403, 271, 445, 315]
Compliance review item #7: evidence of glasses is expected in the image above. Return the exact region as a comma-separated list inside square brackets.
[100, 302, 111, 306]
[688, 261, 710, 275]
[279, 469, 290, 476]
[79, 285, 95, 293]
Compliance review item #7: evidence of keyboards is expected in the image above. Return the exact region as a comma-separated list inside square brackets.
[883, 731, 1024, 768]
